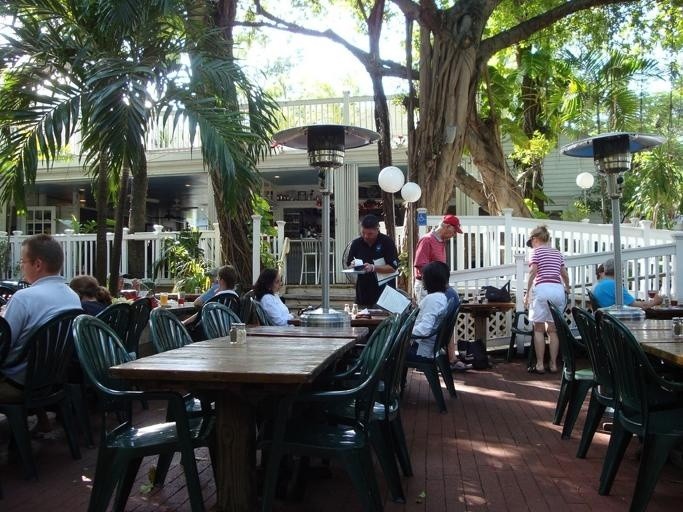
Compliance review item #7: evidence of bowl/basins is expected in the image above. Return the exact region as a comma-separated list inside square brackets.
[671, 301, 678, 306]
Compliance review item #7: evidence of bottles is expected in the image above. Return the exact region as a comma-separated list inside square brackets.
[344, 304, 350, 313]
[276, 194, 296, 201]
[665, 295, 670, 307]
[353, 304, 358, 315]
[230, 322, 247, 344]
[672, 317, 683, 337]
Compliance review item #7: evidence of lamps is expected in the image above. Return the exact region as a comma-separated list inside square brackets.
[272, 122, 383, 328]
[559, 133, 667, 321]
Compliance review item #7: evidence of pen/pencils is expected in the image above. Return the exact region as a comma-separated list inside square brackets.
[351, 256, 360, 264]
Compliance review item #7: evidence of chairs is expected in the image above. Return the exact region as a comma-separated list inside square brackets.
[408, 296, 460, 413]
[299, 234, 318, 285]
[545, 298, 682, 512]
[317, 236, 336, 284]
[0, 281, 421, 512]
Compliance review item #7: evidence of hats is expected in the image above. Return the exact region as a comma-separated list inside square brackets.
[442, 214, 463, 235]
[526, 230, 539, 249]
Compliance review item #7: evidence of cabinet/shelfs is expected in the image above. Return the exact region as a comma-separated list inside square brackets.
[283, 211, 304, 239]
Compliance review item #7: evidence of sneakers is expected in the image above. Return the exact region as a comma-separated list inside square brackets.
[451, 360, 474, 370]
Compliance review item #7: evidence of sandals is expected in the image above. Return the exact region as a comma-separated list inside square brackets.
[547, 363, 559, 374]
[528, 364, 545, 374]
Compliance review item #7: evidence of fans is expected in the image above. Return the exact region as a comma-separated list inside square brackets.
[166, 198, 199, 210]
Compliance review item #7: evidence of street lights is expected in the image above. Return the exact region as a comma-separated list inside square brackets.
[400, 180, 423, 294]
[575, 170, 597, 215]
[376, 165, 405, 247]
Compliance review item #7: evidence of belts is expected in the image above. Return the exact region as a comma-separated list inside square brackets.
[0, 372, 24, 390]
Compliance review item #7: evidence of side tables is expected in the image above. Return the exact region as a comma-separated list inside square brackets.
[458, 301, 516, 369]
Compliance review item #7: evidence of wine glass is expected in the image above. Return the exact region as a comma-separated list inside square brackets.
[647, 290, 657, 298]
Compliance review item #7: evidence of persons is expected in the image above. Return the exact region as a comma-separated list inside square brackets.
[414, 215, 473, 370]
[346, 215, 399, 309]
[181, 265, 239, 326]
[375, 261, 448, 409]
[588, 264, 605, 309]
[70, 276, 112, 325]
[254, 269, 298, 327]
[0, 235, 82, 407]
[592, 258, 663, 310]
[522, 226, 570, 373]
[434, 261, 459, 318]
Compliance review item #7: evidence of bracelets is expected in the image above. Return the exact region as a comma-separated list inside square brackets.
[371, 264, 374, 272]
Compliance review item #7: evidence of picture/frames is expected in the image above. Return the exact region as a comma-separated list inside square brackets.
[298, 191, 306, 201]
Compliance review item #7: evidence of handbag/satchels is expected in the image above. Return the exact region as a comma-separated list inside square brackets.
[484, 279, 512, 303]
[457, 339, 488, 368]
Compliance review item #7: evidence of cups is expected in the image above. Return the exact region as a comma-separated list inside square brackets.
[178, 291, 185, 304]
[159, 292, 168, 305]
